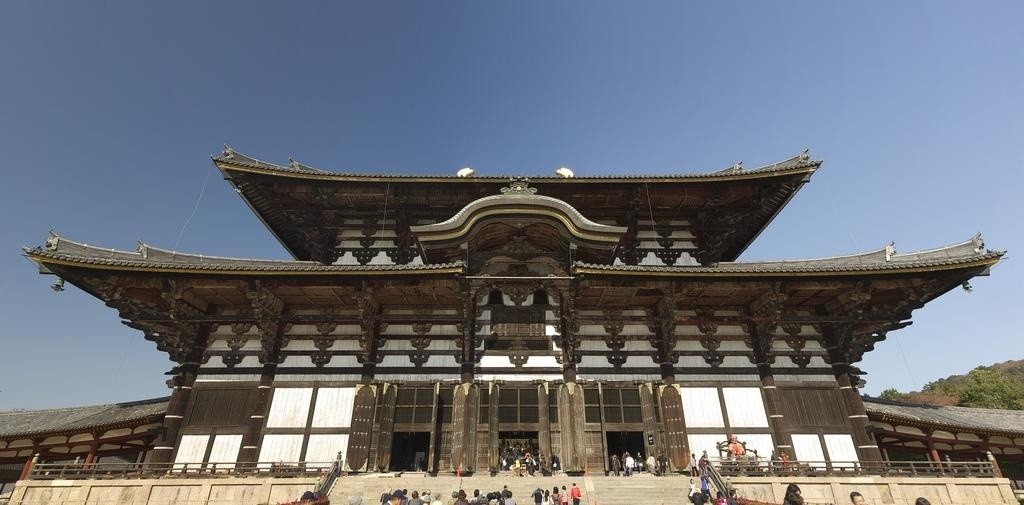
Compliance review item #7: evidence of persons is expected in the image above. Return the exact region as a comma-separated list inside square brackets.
[378, 481, 582, 505]
[790, 492, 803, 505]
[314, 496, 330, 505]
[416, 452, 424, 472]
[850, 491, 866, 505]
[782, 483, 802, 505]
[498, 437, 558, 477]
[299, 491, 315, 505]
[609, 450, 780, 505]
